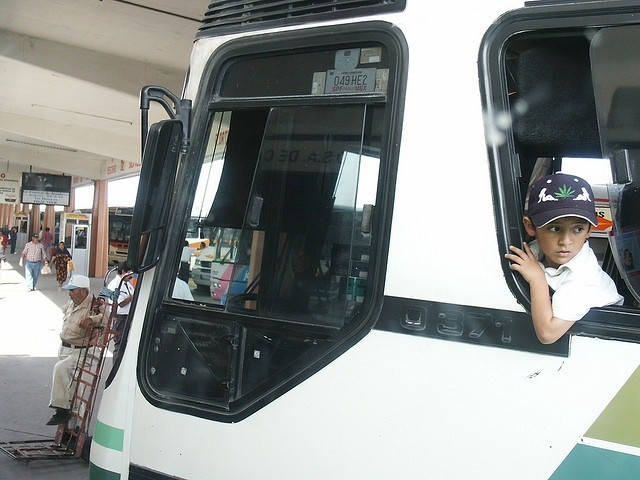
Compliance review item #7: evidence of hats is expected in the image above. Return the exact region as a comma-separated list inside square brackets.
[61, 274, 90, 290]
[528, 173, 599, 229]
[32, 234, 39, 238]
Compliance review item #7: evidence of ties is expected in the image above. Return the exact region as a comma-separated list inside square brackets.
[125, 282, 132, 296]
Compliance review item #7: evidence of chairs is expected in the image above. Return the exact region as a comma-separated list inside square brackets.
[590, 184, 636, 297]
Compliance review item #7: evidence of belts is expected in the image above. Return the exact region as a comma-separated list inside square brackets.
[61, 341, 94, 349]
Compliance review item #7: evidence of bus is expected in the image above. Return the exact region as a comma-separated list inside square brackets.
[87, 0, 640, 480]
[210, 225, 242, 304]
[178, 217, 207, 280]
[74, 207, 135, 266]
[192, 218, 245, 289]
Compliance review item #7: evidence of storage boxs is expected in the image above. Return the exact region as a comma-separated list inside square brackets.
[339, 277, 367, 303]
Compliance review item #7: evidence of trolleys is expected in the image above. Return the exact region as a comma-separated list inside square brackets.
[0, 266, 134, 466]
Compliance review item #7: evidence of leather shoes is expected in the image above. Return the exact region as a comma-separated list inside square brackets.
[46, 408, 73, 426]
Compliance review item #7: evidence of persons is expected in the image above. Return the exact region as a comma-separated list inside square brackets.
[502, 171, 622, 342]
[109, 266, 139, 375]
[19, 234, 48, 292]
[10, 228, 17, 254]
[179, 241, 195, 282]
[197, 242, 206, 252]
[42, 228, 53, 250]
[46, 275, 107, 427]
[39, 226, 43, 241]
[171, 276, 193, 302]
[51, 242, 72, 290]
[4, 225, 9, 234]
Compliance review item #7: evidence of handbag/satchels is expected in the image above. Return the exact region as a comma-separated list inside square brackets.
[67, 261, 74, 272]
